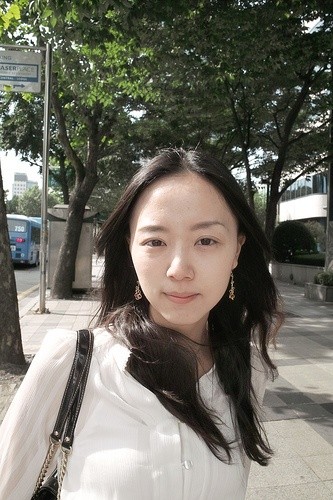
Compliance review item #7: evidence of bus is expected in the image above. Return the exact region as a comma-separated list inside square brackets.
[5, 212, 42, 268]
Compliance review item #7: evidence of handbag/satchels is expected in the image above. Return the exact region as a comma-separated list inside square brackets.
[30, 329, 94, 500]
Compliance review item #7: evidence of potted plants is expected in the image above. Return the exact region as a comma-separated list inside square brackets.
[305, 272, 333, 302]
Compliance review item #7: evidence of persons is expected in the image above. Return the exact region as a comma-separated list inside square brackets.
[0, 148, 284, 500]
